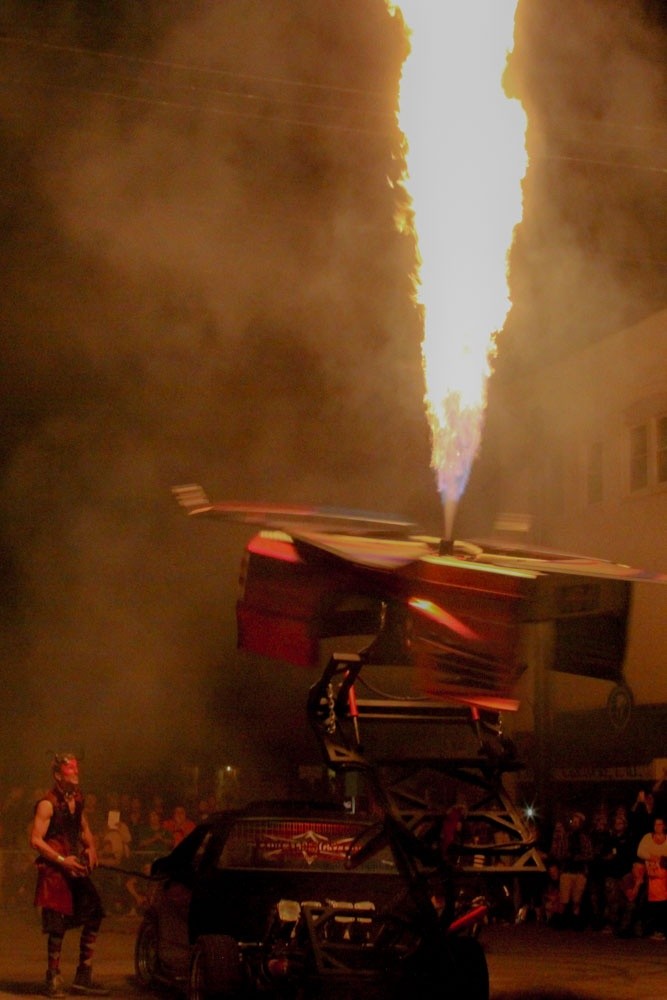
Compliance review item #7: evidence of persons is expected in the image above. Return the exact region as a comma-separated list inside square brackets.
[501, 802, 667, 940]
[31, 754, 108, 998]
[0, 783, 233, 918]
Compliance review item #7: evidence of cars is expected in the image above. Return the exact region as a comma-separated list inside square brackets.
[134, 800, 490, 1000]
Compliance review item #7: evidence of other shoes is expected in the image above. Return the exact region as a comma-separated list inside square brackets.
[42, 976, 66, 998]
[72, 979, 111, 995]
[649, 930, 666, 940]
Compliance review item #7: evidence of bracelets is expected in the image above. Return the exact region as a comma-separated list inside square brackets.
[57, 856, 64, 865]
